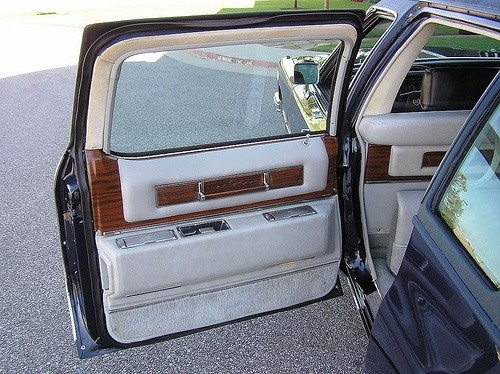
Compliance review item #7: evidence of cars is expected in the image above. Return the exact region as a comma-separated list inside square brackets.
[55, 1, 500, 373]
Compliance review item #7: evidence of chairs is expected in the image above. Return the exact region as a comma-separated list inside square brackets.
[419, 65, 495, 111]
